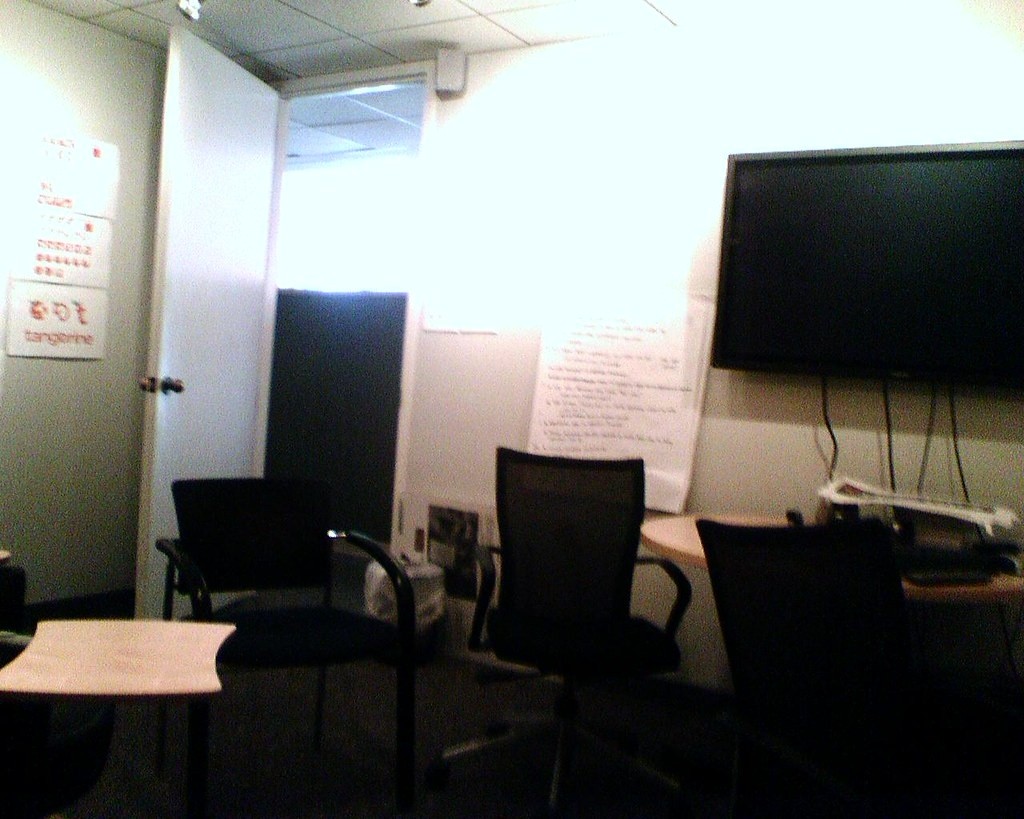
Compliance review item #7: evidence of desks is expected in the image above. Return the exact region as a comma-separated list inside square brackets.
[0, 609, 239, 717]
[635, 502, 1023, 621]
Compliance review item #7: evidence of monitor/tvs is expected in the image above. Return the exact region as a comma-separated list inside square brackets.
[709, 138, 1024, 392]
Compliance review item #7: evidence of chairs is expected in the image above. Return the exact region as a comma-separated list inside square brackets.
[0, 445, 1023, 819]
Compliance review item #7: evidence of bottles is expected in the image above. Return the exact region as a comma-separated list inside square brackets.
[0, 551, 26, 627]
